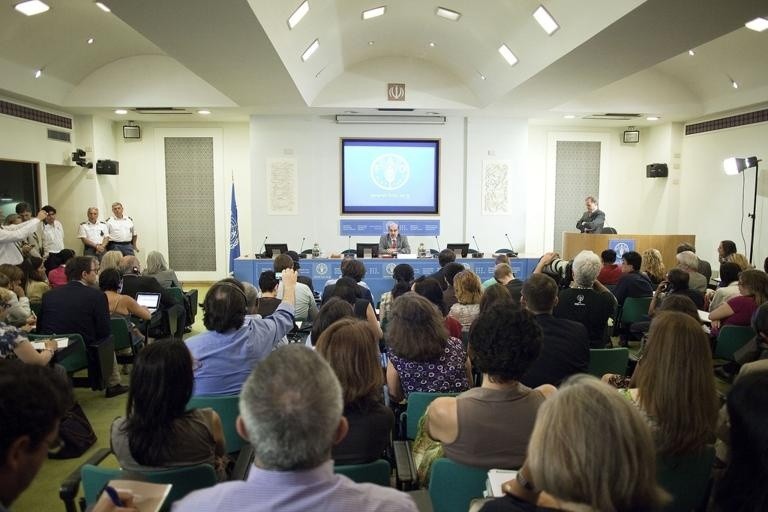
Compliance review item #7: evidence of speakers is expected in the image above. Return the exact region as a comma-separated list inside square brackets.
[96, 160, 120, 176]
[646, 163, 668, 178]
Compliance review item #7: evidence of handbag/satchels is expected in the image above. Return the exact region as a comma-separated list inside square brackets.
[50, 401, 97, 461]
[412, 417, 443, 491]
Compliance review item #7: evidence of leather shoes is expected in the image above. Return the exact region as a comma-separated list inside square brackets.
[105, 384, 129, 398]
[714, 364, 734, 384]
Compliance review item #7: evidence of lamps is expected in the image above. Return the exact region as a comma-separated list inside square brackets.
[623, 126, 640, 143]
[724, 154, 760, 266]
[122, 120, 141, 139]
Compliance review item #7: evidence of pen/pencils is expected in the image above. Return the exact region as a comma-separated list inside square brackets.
[104, 484, 124, 507]
[48, 332, 57, 343]
[31, 310, 37, 319]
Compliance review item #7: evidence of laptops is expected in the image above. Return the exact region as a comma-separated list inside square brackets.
[134, 292, 161, 314]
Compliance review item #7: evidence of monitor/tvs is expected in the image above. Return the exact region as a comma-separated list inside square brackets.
[357, 242, 379, 259]
[264, 243, 288, 260]
[447, 243, 470, 258]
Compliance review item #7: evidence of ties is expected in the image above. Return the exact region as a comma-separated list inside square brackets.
[393, 240, 396, 248]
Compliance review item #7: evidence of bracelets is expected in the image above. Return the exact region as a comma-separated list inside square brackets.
[102, 245, 106, 248]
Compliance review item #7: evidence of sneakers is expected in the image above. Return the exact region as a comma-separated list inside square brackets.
[122, 368, 128, 375]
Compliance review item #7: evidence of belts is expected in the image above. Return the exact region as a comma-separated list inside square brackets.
[109, 240, 132, 246]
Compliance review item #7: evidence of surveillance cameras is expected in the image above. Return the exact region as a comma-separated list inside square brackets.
[71, 149, 93, 169]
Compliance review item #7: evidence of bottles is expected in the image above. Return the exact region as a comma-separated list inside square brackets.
[311, 242, 322, 260]
[416, 243, 427, 259]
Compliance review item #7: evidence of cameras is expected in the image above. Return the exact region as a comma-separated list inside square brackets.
[275, 272, 283, 280]
[542, 258, 574, 290]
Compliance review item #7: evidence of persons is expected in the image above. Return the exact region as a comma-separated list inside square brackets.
[105, 202, 140, 257]
[0, 207, 48, 271]
[377, 222, 411, 255]
[575, 196, 605, 234]
[5, 214, 32, 266]
[1, 244, 192, 511]
[16, 202, 50, 266]
[41, 205, 64, 264]
[77, 207, 109, 266]
[112, 240, 767, 511]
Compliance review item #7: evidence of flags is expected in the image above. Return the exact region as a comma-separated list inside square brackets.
[229, 182, 239, 272]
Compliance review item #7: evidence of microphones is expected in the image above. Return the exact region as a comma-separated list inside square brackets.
[348, 235, 351, 250]
[505, 233, 514, 250]
[388, 233, 389, 236]
[435, 235, 441, 251]
[301, 238, 305, 252]
[472, 235, 481, 257]
[255, 236, 267, 257]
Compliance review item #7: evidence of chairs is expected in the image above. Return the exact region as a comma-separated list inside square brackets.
[301, 249, 513, 254]
